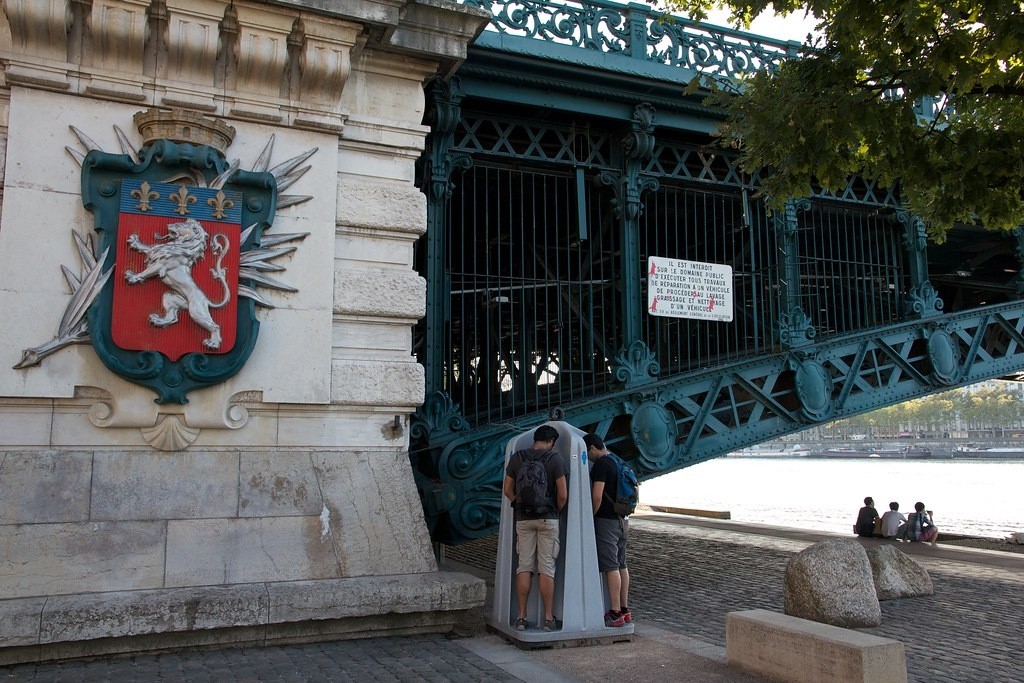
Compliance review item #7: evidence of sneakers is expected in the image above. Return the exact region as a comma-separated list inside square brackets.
[605, 612, 626, 627]
[623, 610, 632, 623]
[544, 615, 562, 632]
[516, 617, 528, 631]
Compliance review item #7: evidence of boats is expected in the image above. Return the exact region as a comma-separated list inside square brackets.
[820, 448, 932, 458]
[727, 448, 811, 458]
[933, 442, 1024, 459]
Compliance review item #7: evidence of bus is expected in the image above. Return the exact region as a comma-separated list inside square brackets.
[899, 432, 926, 439]
[852, 434, 874, 440]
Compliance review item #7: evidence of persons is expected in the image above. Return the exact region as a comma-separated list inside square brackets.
[856, 497, 880, 537]
[504, 425, 567, 632]
[881, 502, 908, 538]
[908, 502, 938, 546]
[583, 433, 631, 627]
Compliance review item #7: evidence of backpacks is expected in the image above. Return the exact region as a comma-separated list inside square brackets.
[596, 453, 639, 529]
[511, 450, 560, 515]
